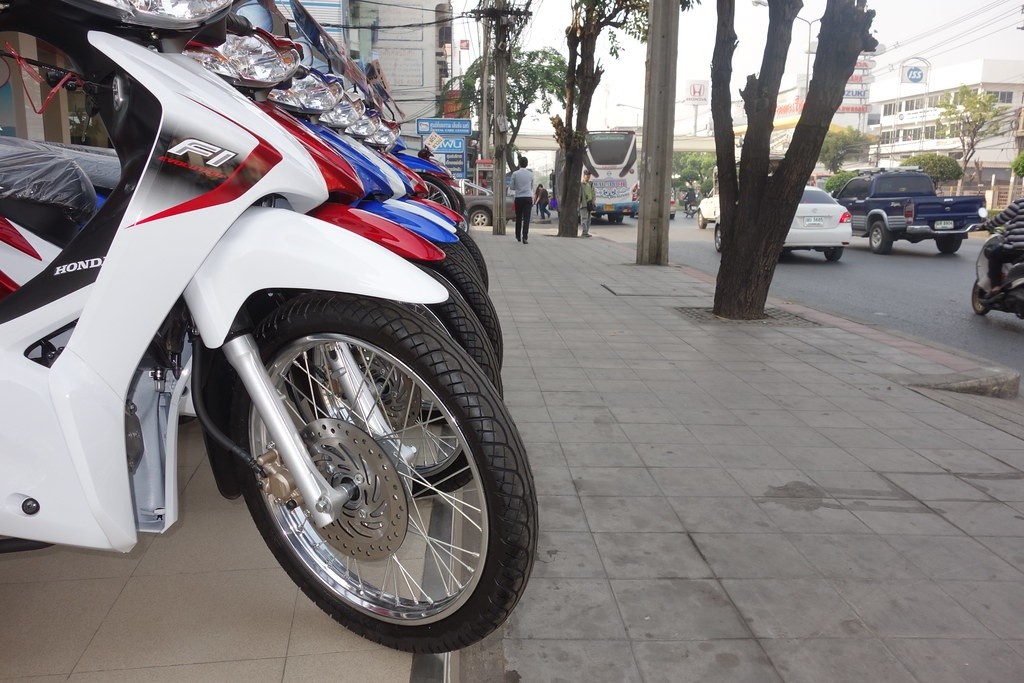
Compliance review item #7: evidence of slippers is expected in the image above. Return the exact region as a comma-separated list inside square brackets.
[983, 288, 1002, 299]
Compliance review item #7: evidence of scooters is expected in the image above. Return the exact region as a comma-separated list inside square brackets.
[971, 206, 1024, 319]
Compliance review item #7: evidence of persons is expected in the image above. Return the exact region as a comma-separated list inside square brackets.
[509, 157, 535, 244]
[684, 188, 695, 212]
[535, 184, 551, 219]
[976, 197, 1024, 299]
[577, 169, 596, 237]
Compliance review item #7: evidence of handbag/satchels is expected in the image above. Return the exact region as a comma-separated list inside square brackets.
[587, 200, 596, 211]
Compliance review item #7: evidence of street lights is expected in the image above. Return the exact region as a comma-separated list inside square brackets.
[752, 0, 822, 95]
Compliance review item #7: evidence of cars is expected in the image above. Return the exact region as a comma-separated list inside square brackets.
[713, 185, 854, 261]
[452, 178, 516, 226]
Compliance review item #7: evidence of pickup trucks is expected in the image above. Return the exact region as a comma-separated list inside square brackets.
[831, 172, 987, 255]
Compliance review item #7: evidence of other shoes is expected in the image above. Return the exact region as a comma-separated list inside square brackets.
[540, 217, 545, 219]
[548, 213, 551, 218]
[516, 236, 521, 242]
[582, 233, 592, 237]
[523, 239, 528, 243]
[684, 210, 688, 214]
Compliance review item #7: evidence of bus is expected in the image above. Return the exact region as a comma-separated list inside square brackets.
[549, 129, 640, 225]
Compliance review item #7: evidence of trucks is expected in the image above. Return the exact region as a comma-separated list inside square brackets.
[697, 165, 740, 230]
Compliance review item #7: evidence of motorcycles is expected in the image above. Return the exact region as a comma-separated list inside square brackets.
[0, 1, 541, 655]
[683, 198, 698, 219]
[1, 1, 505, 498]
